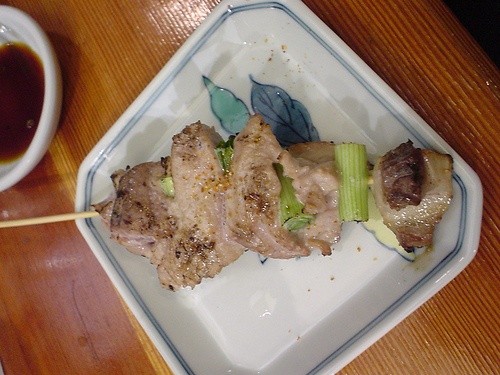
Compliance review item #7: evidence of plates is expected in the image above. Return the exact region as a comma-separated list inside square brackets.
[74, 0, 483, 374]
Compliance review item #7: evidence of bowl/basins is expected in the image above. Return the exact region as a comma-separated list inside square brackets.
[0, 3, 63, 197]
